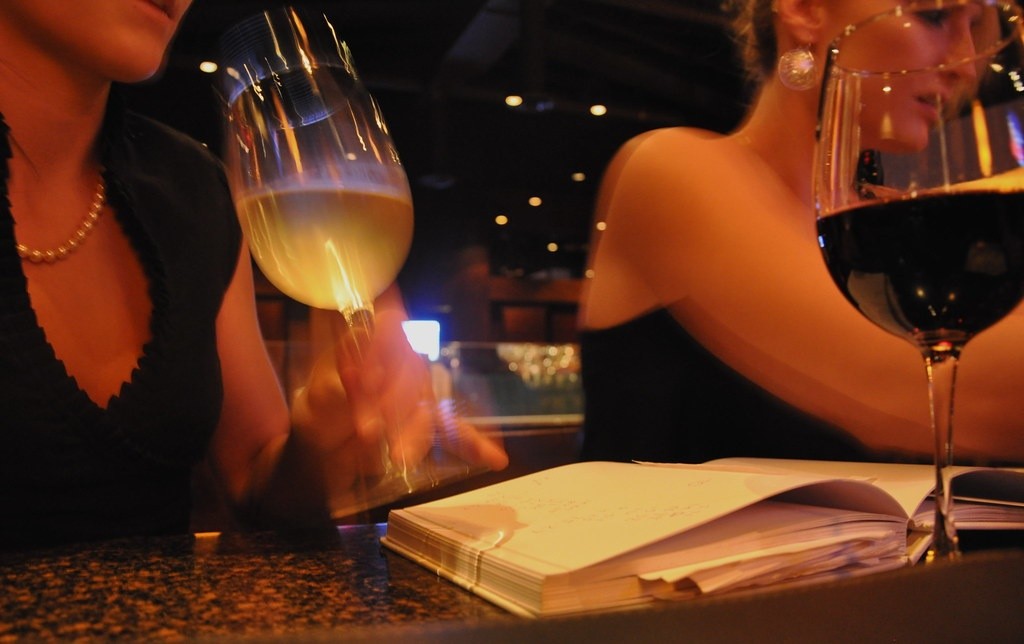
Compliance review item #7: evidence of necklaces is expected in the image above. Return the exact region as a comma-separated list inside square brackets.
[15, 169, 104, 264]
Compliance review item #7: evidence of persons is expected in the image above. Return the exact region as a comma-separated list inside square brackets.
[0, 0, 437, 547]
[575, 1, 1024, 462]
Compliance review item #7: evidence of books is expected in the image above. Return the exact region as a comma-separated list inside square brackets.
[378, 454, 1024, 617]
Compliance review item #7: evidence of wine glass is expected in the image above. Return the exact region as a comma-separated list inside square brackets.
[809, 0, 1024, 568]
[215, 5, 496, 521]
